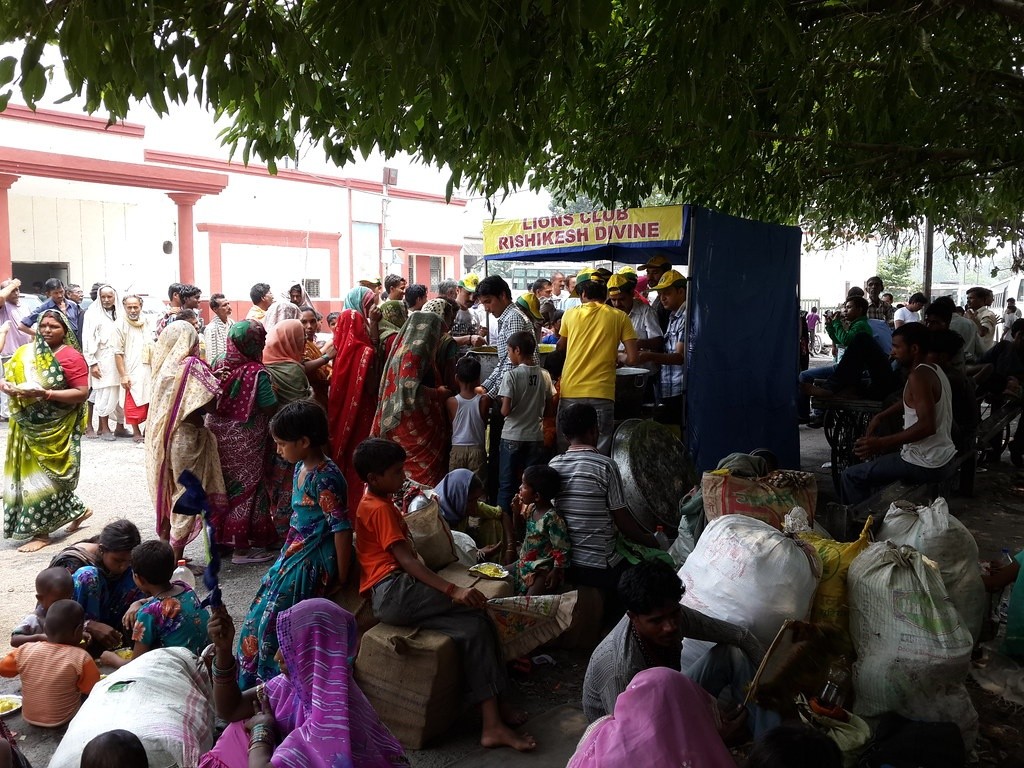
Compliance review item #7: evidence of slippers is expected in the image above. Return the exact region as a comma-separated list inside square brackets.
[232, 547, 274, 564]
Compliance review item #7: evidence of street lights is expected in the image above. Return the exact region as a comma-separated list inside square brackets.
[379, 167, 400, 281]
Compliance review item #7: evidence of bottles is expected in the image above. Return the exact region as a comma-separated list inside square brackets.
[170, 560, 195, 590]
[1001, 549, 1013, 600]
[654, 526, 670, 550]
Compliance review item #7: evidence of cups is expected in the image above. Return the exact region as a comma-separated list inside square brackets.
[818, 680, 847, 708]
[853, 443, 873, 463]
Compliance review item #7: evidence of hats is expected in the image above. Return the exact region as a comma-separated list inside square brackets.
[568, 268, 606, 298]
[517, 293, 545, 319]
[457, 272, 480, 292]
[650, 270, 685, 290]
[356, 265, 381, 284]
[607, 273, 631, 295]
[616, 266, 639, 284]
[637, 254, 672, 271]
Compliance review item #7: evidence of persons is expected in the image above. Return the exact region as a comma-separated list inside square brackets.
[564, 559, 847, 768]
[1, 520, 412, 768]
[989, 547, 1024, 667]
[0, 273, 159, 554]
[158, 256, 687, 750]
[806, 276, 1024, 541]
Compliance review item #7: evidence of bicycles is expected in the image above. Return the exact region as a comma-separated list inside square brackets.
[808, 322, 822, 356]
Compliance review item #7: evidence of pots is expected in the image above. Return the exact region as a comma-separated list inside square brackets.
[611, 418, 699, 540]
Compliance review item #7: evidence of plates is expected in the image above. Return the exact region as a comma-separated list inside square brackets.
[0, 695, 23, 715]
[468, 562, 510, 579]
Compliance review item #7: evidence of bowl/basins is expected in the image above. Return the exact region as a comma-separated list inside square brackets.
[537, 344, 557, 368]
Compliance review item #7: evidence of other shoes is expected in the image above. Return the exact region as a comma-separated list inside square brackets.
[102, 432, 116, 441]
[115, 428, 134, 438]
[807, 416, 835, 430]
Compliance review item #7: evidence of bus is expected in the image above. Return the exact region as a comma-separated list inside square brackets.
[508, 261, 613, 303]
[986, 272, 1024, 343]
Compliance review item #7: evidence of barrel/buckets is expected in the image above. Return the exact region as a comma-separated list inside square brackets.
[467, 346, 500, 385]
[615, 367, 650, 420]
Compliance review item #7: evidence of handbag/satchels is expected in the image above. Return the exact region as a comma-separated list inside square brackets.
[393, 479, 459, 572]
